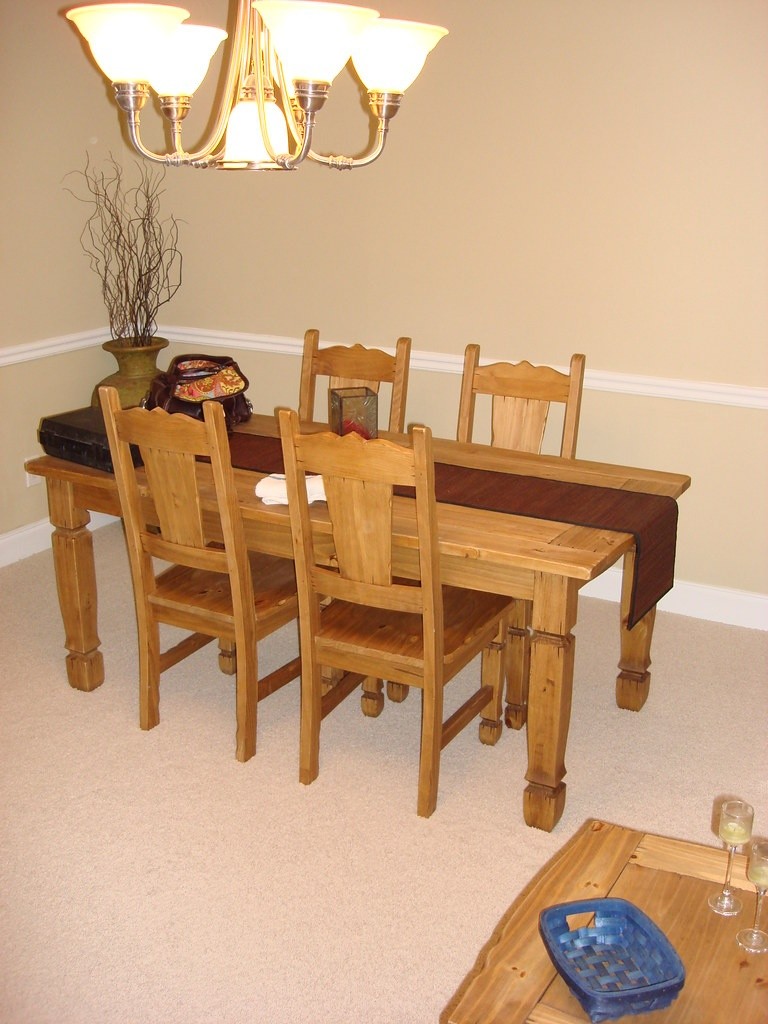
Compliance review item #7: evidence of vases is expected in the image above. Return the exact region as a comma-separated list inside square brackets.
[330, 386, 378, 439]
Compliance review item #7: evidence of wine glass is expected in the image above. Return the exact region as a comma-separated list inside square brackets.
[737, 841, 768, 954]
[709, 800, 754, 915]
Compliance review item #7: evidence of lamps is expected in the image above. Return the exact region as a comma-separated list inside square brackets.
[66, 0, 450, 172]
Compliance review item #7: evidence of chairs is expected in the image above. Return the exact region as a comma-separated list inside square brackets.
[386, 343, 586, 731]
[98, 385, 343, 764]
[278, 409, 516, 819]
[298, 329, 411, 434]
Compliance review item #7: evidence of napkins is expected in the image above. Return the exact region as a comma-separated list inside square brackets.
[255, 472, 329, 506]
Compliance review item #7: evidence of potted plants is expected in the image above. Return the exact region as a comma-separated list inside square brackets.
[63, 149, 188, 412]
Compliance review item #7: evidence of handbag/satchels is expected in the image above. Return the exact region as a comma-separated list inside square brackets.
[140, 354, 254, 430]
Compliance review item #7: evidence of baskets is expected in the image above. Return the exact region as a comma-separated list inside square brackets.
[539, 897, 685, 1024]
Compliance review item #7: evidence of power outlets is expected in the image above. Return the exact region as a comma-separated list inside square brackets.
[25, 455, 42, 487]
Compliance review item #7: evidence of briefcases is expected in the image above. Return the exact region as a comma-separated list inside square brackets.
[38, 405, 145, 474]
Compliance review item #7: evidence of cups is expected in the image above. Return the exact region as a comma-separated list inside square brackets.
[332, 388, 378, 439]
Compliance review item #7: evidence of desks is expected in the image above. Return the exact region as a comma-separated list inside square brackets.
[437, 817, 768, 1024]
[24, 414, 691, 835]
